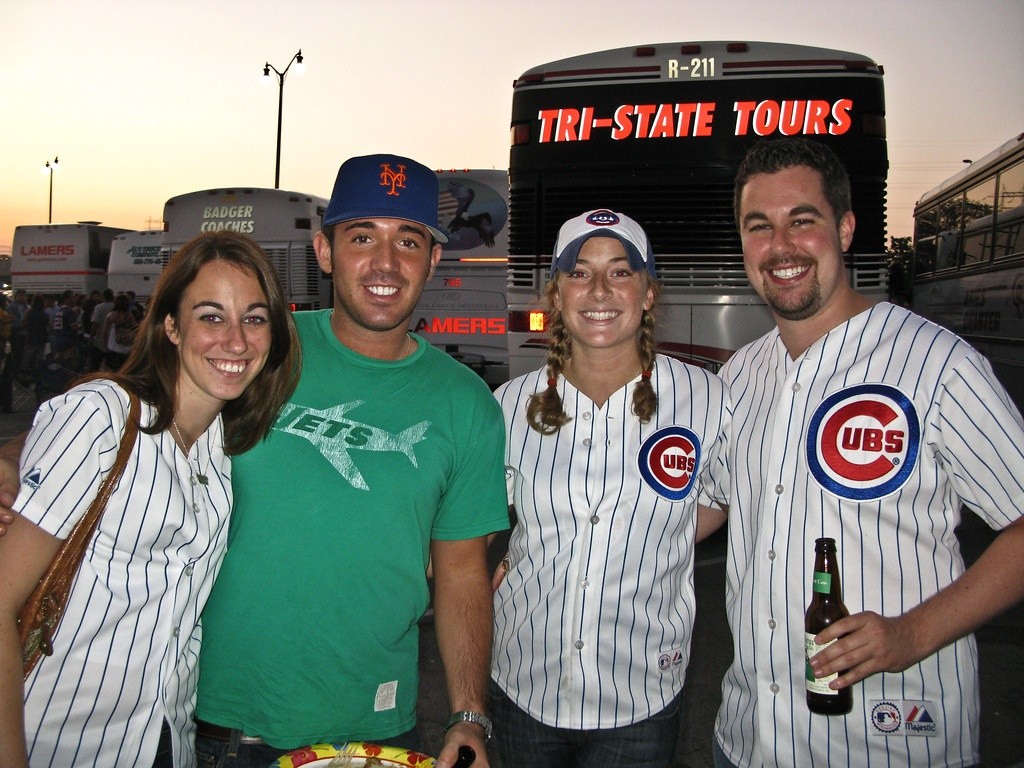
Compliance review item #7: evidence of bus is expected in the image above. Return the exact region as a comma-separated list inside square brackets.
[904, 131, 1024, 419]
[10, 222, 139, 302]
[105, 230, 163, 308]
[505, 38, 890, 383]
[157, 187, 335, 314]
[402, 168, 511, 389]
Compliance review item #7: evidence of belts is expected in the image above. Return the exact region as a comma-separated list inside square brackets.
[193, 717, 266, 745]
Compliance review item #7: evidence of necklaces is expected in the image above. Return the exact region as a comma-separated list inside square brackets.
[404, 330, 412, 356]
[568, 359, 642, 390]
[172, 419, 219, 485]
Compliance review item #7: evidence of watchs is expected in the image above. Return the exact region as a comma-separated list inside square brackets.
[441, 710, 493, 742]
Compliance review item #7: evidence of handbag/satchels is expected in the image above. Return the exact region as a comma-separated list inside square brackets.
[15, 374, 142, 675]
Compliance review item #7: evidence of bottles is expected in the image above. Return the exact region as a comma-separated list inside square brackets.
[805, 539, 853, 716]
[452, 745, 476, 768]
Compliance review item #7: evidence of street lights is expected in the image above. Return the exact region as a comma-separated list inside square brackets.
[263, 47, 304, 189]
[45, 157, 60, 224]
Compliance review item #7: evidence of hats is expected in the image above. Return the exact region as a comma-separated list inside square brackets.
[321, 154, 448, 244]
[549, 208, 656, 282]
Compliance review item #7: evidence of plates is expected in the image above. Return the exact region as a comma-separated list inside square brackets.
[268, 743, 437, 768]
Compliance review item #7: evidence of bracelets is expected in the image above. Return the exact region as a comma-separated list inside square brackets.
[503, 555, 511, 570]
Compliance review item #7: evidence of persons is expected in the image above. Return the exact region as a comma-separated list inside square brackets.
[428, 208, 733, 768]
[0, 282, 324, 414]
[494, 136, 1024, 768]
[0, 233, 301, 768]
[1, 155, 512, 768]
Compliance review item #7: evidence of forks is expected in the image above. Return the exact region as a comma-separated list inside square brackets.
[328, 742, 358, 768]
